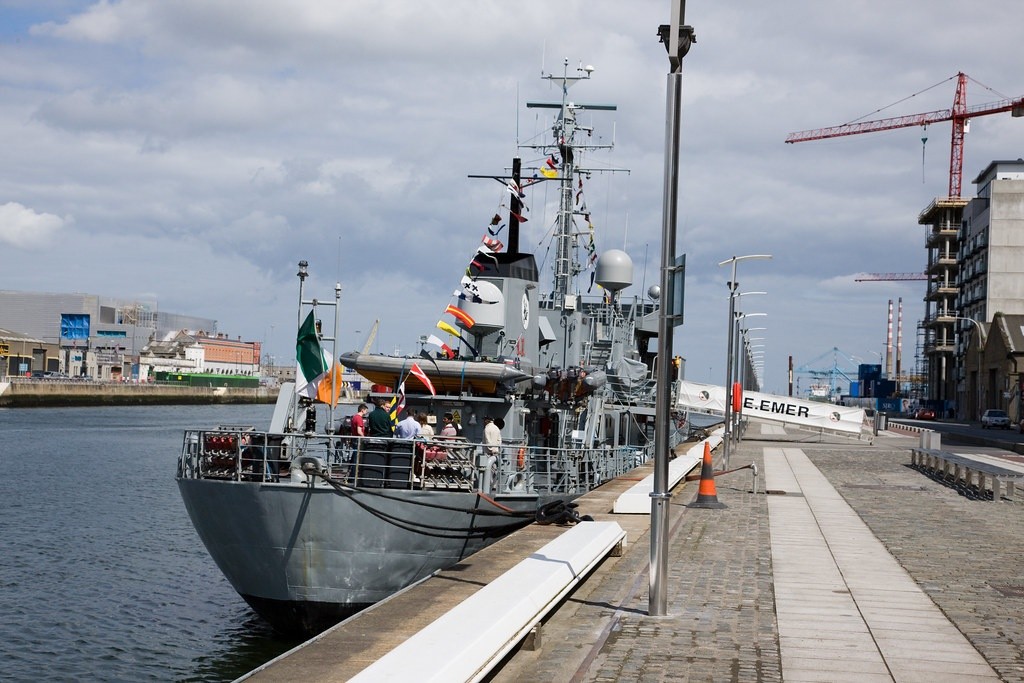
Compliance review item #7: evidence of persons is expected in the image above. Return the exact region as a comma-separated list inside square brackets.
[439, 413, 456, 442]
[349, 404, 368, 464]
[369, 399, 435, 442]
[902, 400, 908, 411]
[481, 414, 502, 475]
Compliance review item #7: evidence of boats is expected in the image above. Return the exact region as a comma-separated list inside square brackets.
[172, 54, 695, 645]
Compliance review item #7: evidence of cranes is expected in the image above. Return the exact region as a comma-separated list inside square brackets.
[852, 271, 940, 280]
[784, 71, 1024, 201]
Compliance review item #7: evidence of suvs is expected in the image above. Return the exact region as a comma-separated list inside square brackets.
[982, 409, 1010, 431]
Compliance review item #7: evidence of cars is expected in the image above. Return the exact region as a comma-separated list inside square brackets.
[31, 369, 94, 383]
[915, 408, 937, 420]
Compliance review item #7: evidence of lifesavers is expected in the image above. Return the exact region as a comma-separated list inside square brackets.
[519, 449, 524, 470]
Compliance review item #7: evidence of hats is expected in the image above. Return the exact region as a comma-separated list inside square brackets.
[482, 414, 494, 421]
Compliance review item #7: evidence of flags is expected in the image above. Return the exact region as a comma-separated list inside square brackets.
[436, 321, 478, 358]
[446, 304, 475, 328]
[410, 364, 436, 396]
[427, 335, 455, 359]
[295, 309, 342, 408]
[541, 154, 559, 177]
[576, 189, 608, 303]
[387, 380, 405, 434]
[453, 179, 530, 304]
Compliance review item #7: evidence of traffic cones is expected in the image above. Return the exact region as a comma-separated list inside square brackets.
[686, 441, 729, 510]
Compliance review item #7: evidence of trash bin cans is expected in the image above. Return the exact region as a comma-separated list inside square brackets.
[878, 412, 888, 431]
[251, 434, 285, 483]
[387, 440, 414, 489]
[357, 439, 388, 489]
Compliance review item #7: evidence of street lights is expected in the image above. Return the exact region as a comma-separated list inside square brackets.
[945, 315, 981, 424]
[20, 332, 28, 385]
[715, 253, 774, 472]
[108, 338, 115, 384]
